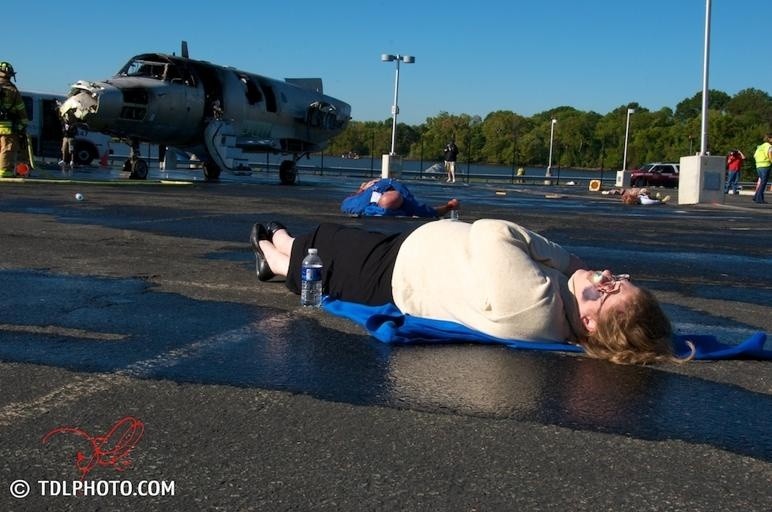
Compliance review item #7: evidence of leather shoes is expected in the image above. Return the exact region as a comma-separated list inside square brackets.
[250, 221, 286, 282]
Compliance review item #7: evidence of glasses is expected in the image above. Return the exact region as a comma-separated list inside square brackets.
[596, 274, 633, 324]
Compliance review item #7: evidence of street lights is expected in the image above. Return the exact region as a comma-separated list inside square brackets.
[378, 50, 417, 158]
[545, 117, 559, 176]
[622, 108, 635, 170]
[687, 135, 697, 154]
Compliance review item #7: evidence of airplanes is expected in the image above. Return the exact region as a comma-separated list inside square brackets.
[57, 37, 355, 186]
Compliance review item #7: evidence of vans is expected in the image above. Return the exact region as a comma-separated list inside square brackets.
[14, 89, 111, 170]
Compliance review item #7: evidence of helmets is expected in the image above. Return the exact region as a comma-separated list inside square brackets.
[0, 62, 15, 76]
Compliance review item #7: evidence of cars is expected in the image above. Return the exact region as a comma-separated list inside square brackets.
[632, 162, 679, 190]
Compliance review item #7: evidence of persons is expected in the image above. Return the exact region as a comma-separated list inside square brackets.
[755, 145, 767, 193]
[723, 148, 747, 195]
[621, 190, 671, 206]
[443, 139, 459, 183]
[249, 218, 697, 368]
[341, 149, 360, 160]
[751, 132, 771, 205]
[601, 187, 650, 197]
[1, 61, 37, 177]
[516, 164, 526, 183]
[57, 121, 78, 169]
[340, 175, 460, 219]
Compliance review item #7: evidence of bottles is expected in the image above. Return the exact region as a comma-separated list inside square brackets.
[75, 193, 84, 201]
[451, 199, 459, 220]
[299, 247, 323, 310]
